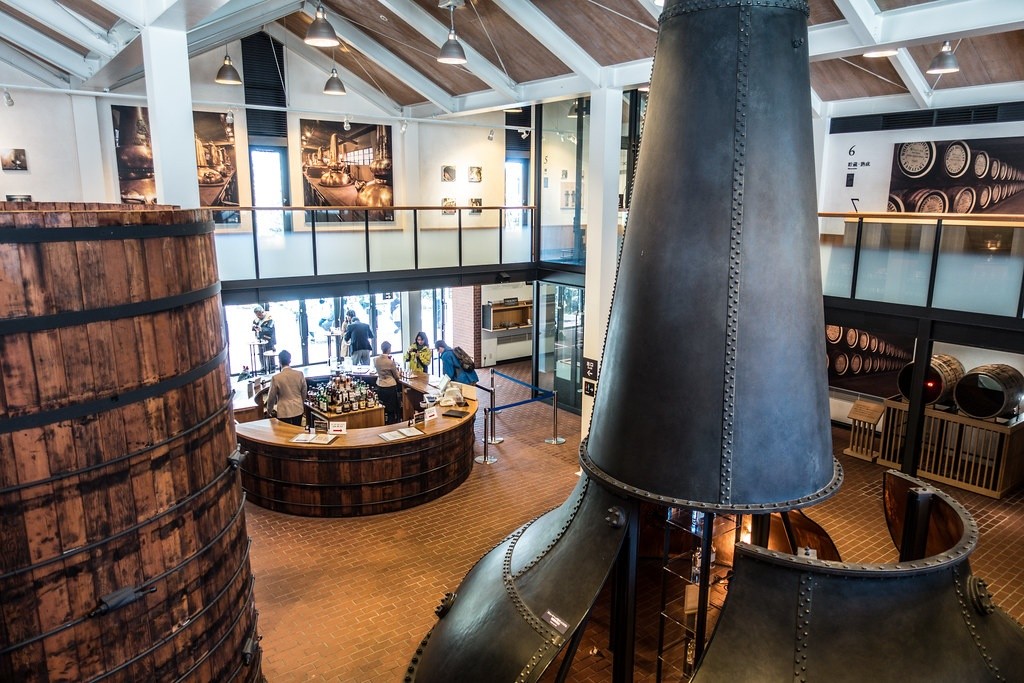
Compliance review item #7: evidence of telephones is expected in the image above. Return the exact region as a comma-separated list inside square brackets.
[440, 397, 456, 406]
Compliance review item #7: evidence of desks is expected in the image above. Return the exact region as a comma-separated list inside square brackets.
[121, 168, 237, 206]
[303, 171, 395, 221]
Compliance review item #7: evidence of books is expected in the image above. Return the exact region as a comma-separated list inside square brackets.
[289, 434, 339, 445]
[378, 426, 426, 442]
[442, 410, 469, 418]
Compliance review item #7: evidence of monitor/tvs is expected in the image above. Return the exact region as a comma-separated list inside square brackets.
[438, 374, 451, 393]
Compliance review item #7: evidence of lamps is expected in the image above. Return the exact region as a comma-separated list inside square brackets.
[4, 93, 14, 106]
[214, 44, 242, 84]
[568, 98, 585, 117]
[925, 41, 961, 74]
[504, 107, 523, 112]
[226, 111, 234, 123]
[303, 7, 339, 46]
[518, 130, 528, 139]
[344, 116, 351, 130]
[401, 121, 407, 132]
[863, 47, 900, 57]
[324, 46, 346, 94]
[437, 0, 467, 64]
[488, 128, 496, 139]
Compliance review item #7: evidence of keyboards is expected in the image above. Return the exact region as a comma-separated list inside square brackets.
[427, 397, 437, 402]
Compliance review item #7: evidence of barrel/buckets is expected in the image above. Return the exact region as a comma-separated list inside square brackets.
[953, 364, 1024, 419]
[825, 324, 911, 377]
[886, 140, 1024, 214]
[896, 354, 966, 406]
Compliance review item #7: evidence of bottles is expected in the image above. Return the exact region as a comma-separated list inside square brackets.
[393, 358, 395, 364]
[308, 373, 379, 414]
[686, 636, 709, 664]
[692, 541, 716, 574]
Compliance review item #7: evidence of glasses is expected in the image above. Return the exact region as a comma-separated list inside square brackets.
[418, 339, 423, 341]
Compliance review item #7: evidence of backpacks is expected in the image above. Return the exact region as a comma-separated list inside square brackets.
[441, 346, 475, 373]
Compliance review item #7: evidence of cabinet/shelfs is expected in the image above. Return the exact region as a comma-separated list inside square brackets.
[302, 397, 385, 429]
[482, 300, 555, 332]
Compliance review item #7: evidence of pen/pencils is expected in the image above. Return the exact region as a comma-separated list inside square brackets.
[464, 397, 475, 401]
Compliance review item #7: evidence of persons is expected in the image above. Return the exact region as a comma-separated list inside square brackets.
[403, 331, 432, 375]
[372, 341, 403, 424]
[341, 310, 356, 358]
[345, 317, 375, 365]
[319, 296, 351, 333]
[265, 350, 307, 426]
[360, 295, 401, 334]
[435, 340, 480, 386]
[294, 307, 316, 342]
[251, 306, 276, 374]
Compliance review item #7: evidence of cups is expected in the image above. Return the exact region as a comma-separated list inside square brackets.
[331, 327, 335, 334]
[249, 331, 255, 343]
[337, 327, 340, 331]
[402, 369, 413, 378]
